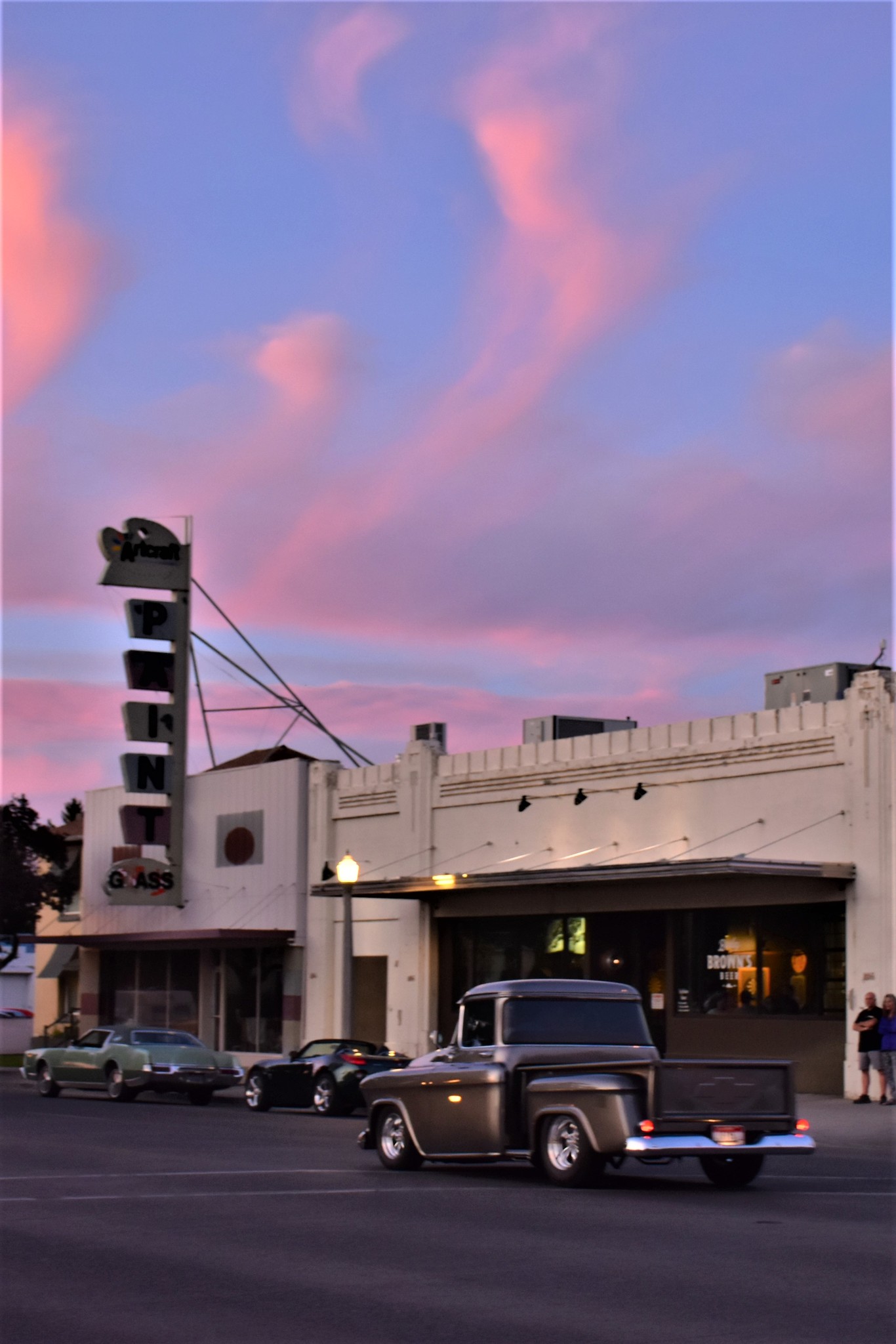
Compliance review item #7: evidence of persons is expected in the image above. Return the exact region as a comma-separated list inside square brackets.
[851, 991, 887, 1105]
[879, 994, 896, 1106]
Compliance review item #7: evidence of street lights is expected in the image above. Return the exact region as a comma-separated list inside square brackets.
[336, 851, 359, 1038]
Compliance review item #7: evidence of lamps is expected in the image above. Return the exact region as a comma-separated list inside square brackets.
[634, 782, 678, 800]
[519, 794, 562, 811]
[322, 856, 370, 880]
[575, 789, 619, 805]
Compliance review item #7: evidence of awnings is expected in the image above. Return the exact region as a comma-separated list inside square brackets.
[310, 810, 859, 897]
[17, 882, 300, 945]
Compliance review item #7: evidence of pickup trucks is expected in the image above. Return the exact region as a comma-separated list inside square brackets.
[356, 979, 816, 1187]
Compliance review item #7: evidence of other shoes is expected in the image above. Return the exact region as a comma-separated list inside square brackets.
[882, 1098, 896, 1105]
[880, 1096, 886, 1104]
[853, 1095, 871, 1104]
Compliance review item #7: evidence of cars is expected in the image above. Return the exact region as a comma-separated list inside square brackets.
[18, 1027, 242, 1103]
[242, 1038, 416, 1116]
